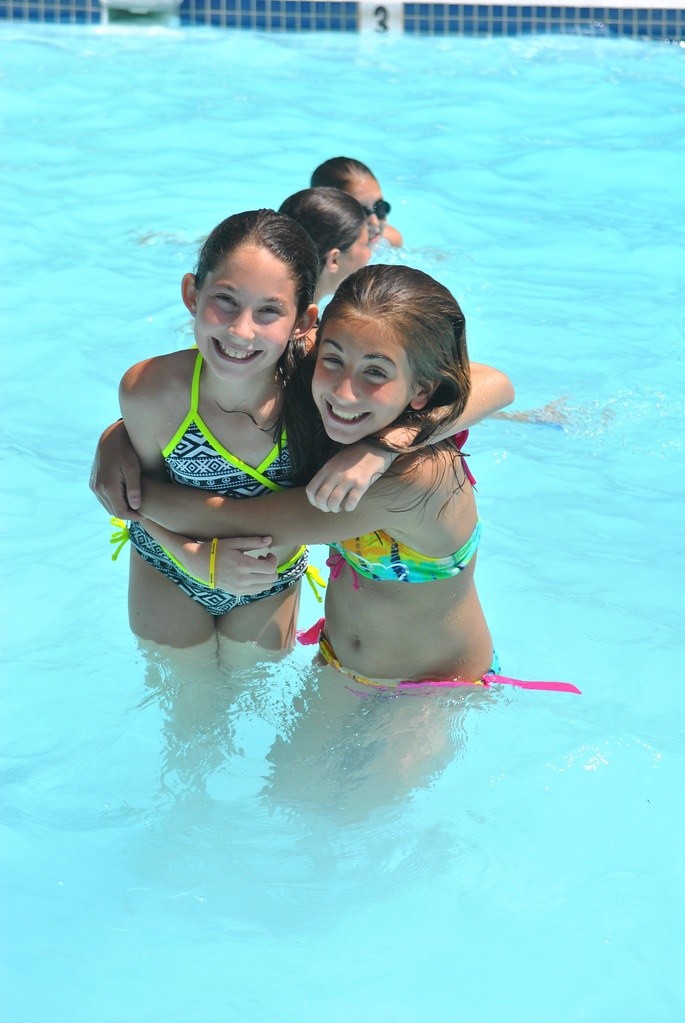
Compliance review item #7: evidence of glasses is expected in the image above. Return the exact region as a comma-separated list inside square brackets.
[364, 200, 390, 220]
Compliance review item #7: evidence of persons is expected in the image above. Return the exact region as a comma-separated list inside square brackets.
[312, 157, 402, 248]
[91, 264, 494, 686]
[117, 208, 515, 650]
[277, 188, 372, 326]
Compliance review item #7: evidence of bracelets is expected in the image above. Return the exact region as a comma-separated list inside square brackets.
[210, 538, 218, 590]
